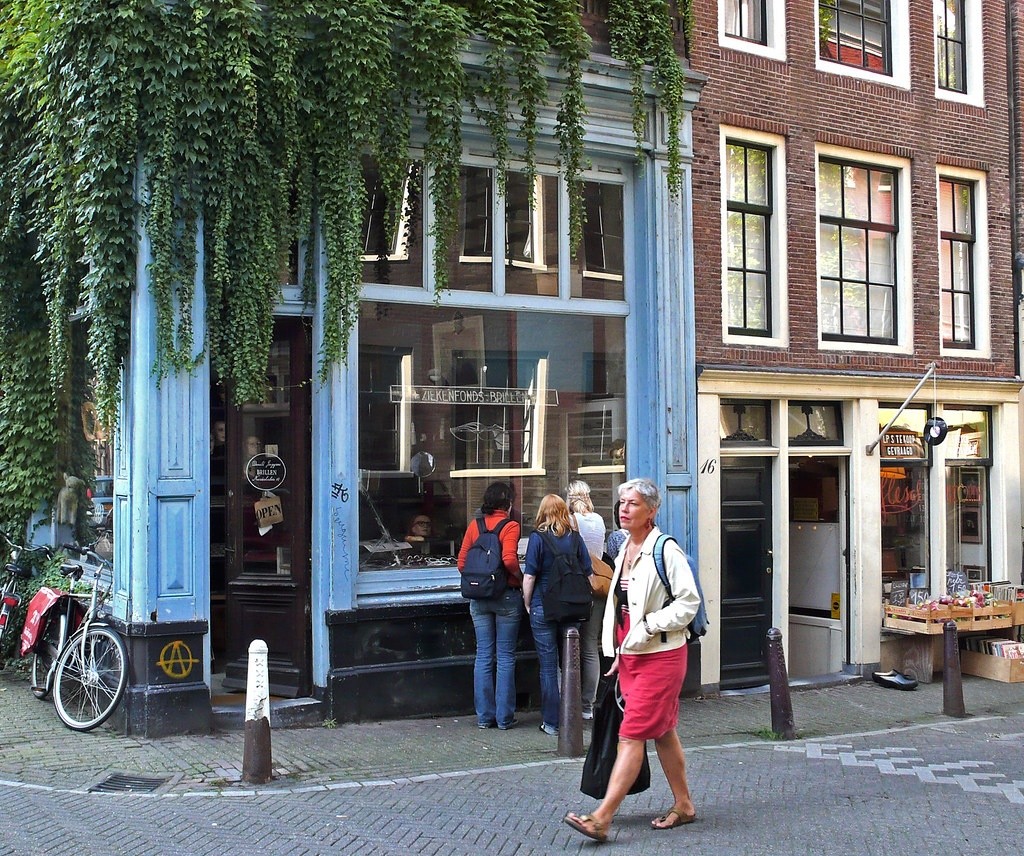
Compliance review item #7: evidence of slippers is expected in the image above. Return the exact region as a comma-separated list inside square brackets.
[649, 806, 695, 829]
[565, 814, 607, 842]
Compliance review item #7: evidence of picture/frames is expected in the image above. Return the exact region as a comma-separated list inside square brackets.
[957, 506, 983, 544]
[963, 564, 986, 585]
[958, 467, 984, 507]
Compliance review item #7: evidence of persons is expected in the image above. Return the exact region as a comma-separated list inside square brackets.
[246, 435, 262, 458]
[412, 512, 432, 536]
[210, 420, 225, 454]
[563, 478, 702, 842]
[522, 493, 593, 734]
[557, 480, 607, 720]
[457, 481, 524, 730]
[607, 501, 630, 564]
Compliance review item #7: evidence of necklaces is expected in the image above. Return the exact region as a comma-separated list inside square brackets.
[627, 540, 644, 569]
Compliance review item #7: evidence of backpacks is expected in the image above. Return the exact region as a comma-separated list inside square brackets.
[529, 530, 593, 624]
[461, 517, 513, 600]
[652, 534, 708, 643]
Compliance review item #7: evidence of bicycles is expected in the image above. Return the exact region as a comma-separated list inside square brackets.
[32, 542, 130, 732]
[0, 530, 54, 647]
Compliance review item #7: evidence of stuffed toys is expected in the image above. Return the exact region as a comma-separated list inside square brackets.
[57, 472, 83, 527]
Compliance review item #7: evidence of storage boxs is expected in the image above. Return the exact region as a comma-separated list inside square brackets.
[884, 598, 1012, 634]
[998, 599, 1024, 626]
[959, 649, 1024, 683]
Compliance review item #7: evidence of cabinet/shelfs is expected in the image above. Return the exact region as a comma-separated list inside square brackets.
[464, 397, 627, 543]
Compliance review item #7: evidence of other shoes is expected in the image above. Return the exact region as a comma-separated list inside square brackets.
[872, 669, 899, 683]
[879, 673, 919, 691]
[500, 719, 518, 729]
[478, 725, 487, 728]
[539, 724, 548, 734]
[582, 712, 593, 720]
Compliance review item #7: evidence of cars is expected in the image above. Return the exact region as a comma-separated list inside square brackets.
[86, 476, 113, 516]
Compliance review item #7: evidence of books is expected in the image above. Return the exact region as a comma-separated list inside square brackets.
[966, 636, 1024, 659]
[969, 580, 1024, 602]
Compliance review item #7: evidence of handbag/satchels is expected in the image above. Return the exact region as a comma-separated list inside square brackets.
[587, 553, 614, 598]
[579, 673, 651, 799]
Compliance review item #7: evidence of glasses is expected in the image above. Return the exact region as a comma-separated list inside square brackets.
[413, 520, 434, 528]
